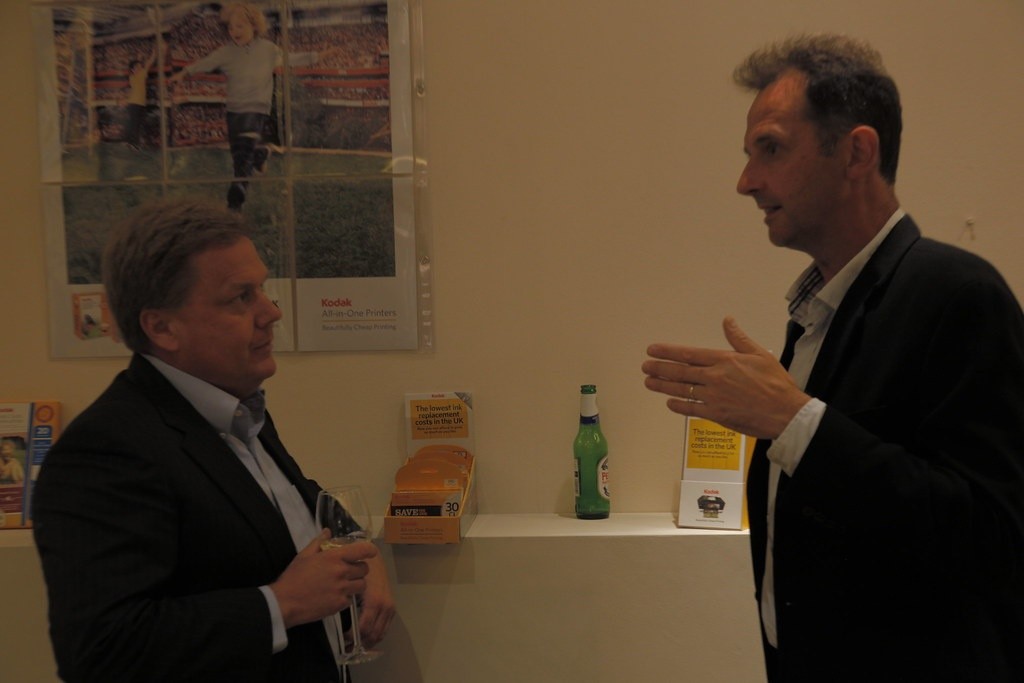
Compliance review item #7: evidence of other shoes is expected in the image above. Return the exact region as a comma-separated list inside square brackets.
[226, 204, 243, 220]
[127, 143, 142, 153]
[251, 145, 271, 180]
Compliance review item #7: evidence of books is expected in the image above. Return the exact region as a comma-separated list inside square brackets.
[0, 399, 62, 529]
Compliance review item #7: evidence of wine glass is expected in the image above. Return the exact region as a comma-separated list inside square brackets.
[316, 486, 382, 664]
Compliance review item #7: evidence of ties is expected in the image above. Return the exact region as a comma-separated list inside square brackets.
[231, 412, 346, 683]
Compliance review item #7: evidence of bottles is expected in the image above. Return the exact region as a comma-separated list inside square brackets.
[573, 384, 609, 519]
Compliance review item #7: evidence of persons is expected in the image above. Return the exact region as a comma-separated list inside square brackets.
[33, 194, 398, 683]
[125, 48, 157, 152]
[641, 34, 1024, 683]
[169, 0, 337, 210]
[1, 442, 23, 479]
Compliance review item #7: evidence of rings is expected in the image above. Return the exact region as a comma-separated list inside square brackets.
[690, 384, 696, 400]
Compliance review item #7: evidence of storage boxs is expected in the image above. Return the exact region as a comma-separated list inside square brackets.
[382, 443, 480, 546]
